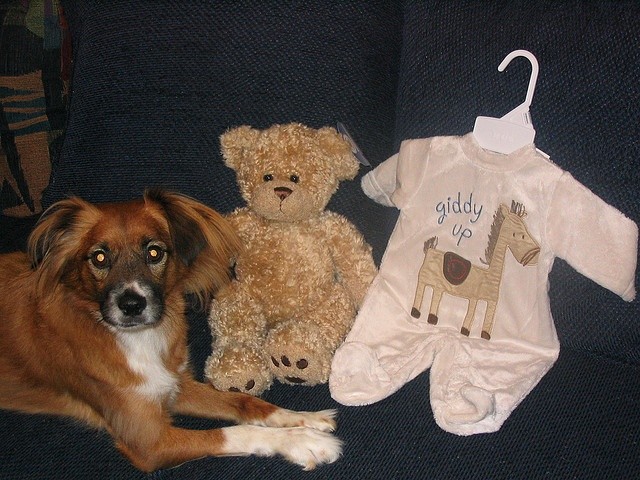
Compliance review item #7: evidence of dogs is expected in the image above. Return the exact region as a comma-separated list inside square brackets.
[0, 187, 348, 474]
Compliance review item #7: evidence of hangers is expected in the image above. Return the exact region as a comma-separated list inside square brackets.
[474, 50, 538, 153]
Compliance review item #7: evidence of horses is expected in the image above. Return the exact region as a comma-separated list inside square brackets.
[411, 200, 540, 340]
